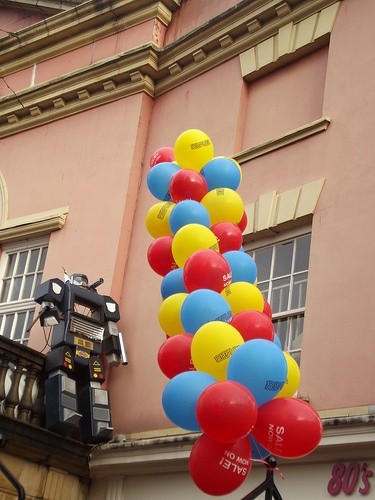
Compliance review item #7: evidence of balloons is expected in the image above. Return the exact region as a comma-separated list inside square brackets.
[200, 187, 244, 227]
[171, 222, 220, 268]
[160, 267, 187, 300]
[149, 147, 174, 168]
[214, 155, 243, 192]
[181, 288, 233, 334]
[210, 221, 243, 254]
[182, 248, 233, 294]
[147, 161, 181, 201]
[237, 211, 248, 233]
[263, 299, 272, 320]
[169, 198, 210, 236]
[144, 200, 176, 239]
[147, 235, 180, 277]
[158, 292, 189, 337]
[169, 169, 209, 204]
[200, 158, 239, 191]
[221, 250, 257, 285]
[173, 129, 214, 173]
[157, 311, 323, 495]
[220, 281, 264, 316]
